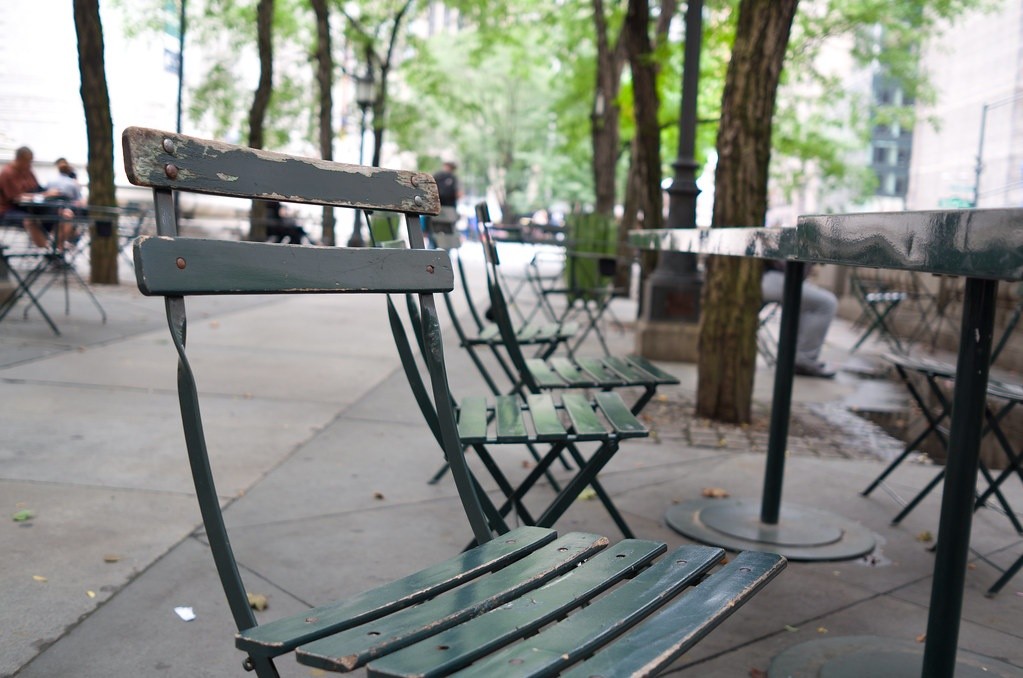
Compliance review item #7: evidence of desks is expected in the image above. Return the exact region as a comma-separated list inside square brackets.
[4, 199, 121, 326]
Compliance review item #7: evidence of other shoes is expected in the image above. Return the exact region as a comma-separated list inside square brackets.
[795, 358, 837, 379]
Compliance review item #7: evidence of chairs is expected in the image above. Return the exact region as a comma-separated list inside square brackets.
[0, 236, 66, 334]
[118, 209, 147, 273]
[753, 263, 1023, 596]
[123, 127, 788, 677]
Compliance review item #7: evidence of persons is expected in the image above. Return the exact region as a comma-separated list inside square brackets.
[0, 147, 65, 268]
[45, 157, 82, 252]
[763, 258, 836, 377]
[426, 161, 457, 249]
